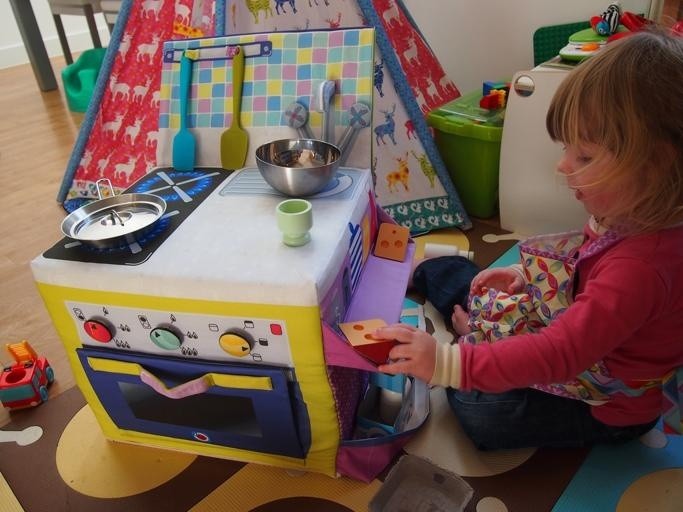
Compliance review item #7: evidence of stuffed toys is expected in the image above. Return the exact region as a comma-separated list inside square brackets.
[592, 5, 621, 36]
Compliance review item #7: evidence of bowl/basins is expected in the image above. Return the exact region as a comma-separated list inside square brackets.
[255, 139, 342, 198]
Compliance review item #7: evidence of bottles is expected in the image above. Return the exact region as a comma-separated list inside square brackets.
[423, 242, 474, 264]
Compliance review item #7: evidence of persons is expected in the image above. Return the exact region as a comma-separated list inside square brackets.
[366, 18, 683, 454]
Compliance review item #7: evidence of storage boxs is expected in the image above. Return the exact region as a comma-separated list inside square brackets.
[424, 80, 512, 219]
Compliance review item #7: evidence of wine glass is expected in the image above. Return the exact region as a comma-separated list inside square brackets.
[275, 199, 314, 247]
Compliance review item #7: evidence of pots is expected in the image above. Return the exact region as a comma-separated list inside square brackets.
[61, 179, 167, 249]
